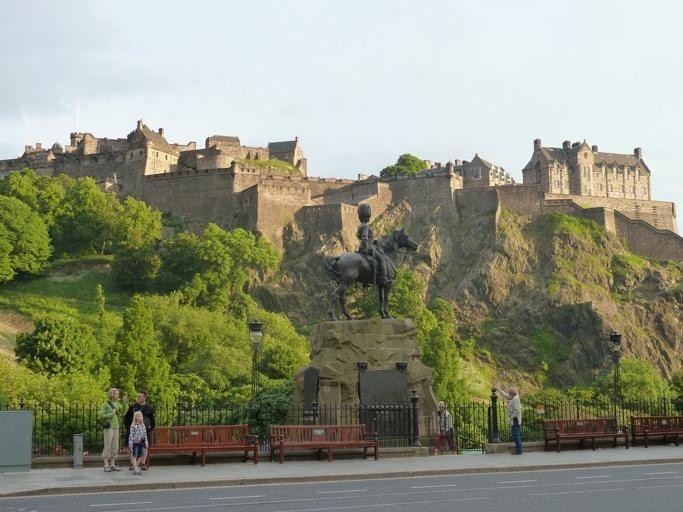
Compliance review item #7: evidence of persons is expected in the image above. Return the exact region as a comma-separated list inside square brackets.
[496, 386, 522, 456]
[354, 204, 392, 287]
[124, 391, 156, 471]
[100, 388, 122, 474]
[128, 412, 148, 476]
[433, 401, 458, 456]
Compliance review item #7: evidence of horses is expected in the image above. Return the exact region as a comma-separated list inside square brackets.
[324, 225, 418, 322]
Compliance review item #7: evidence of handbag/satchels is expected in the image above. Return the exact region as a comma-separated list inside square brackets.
[96, 419, 110, 428]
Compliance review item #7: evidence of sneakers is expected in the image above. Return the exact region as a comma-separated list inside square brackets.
[104, 464, 149, 471]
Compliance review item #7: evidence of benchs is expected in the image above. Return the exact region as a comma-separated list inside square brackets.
[268, 424, 378, 464]
[630, 416, 683, 448]
[146, 424, 259, 468]
[542, 417, 629, 453]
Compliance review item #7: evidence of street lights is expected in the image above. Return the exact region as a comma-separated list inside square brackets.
[609, 332, 624, 437]
[247, 320, 263, 451]
